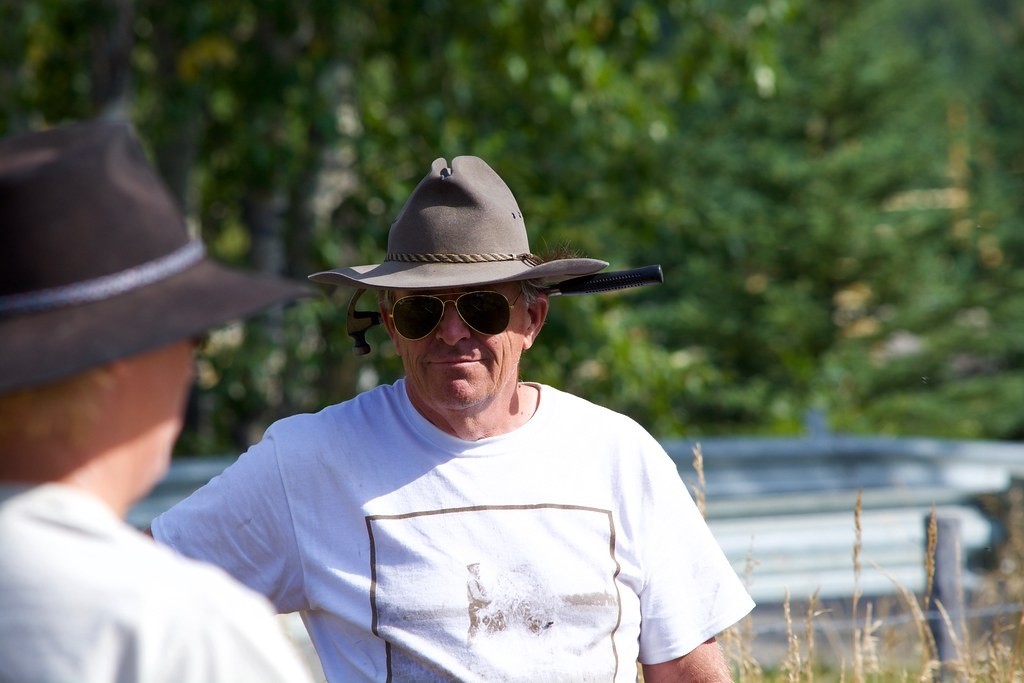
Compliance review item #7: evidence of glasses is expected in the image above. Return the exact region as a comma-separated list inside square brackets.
[388, 290, 523, 340]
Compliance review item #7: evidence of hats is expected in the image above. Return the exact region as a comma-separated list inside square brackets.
[0, 120, 303, 394]
[306, 155, 610, 288]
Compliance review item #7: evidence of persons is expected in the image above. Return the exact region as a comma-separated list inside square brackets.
[0, 115, 318, 683]
[140, 154, 757, 680]
